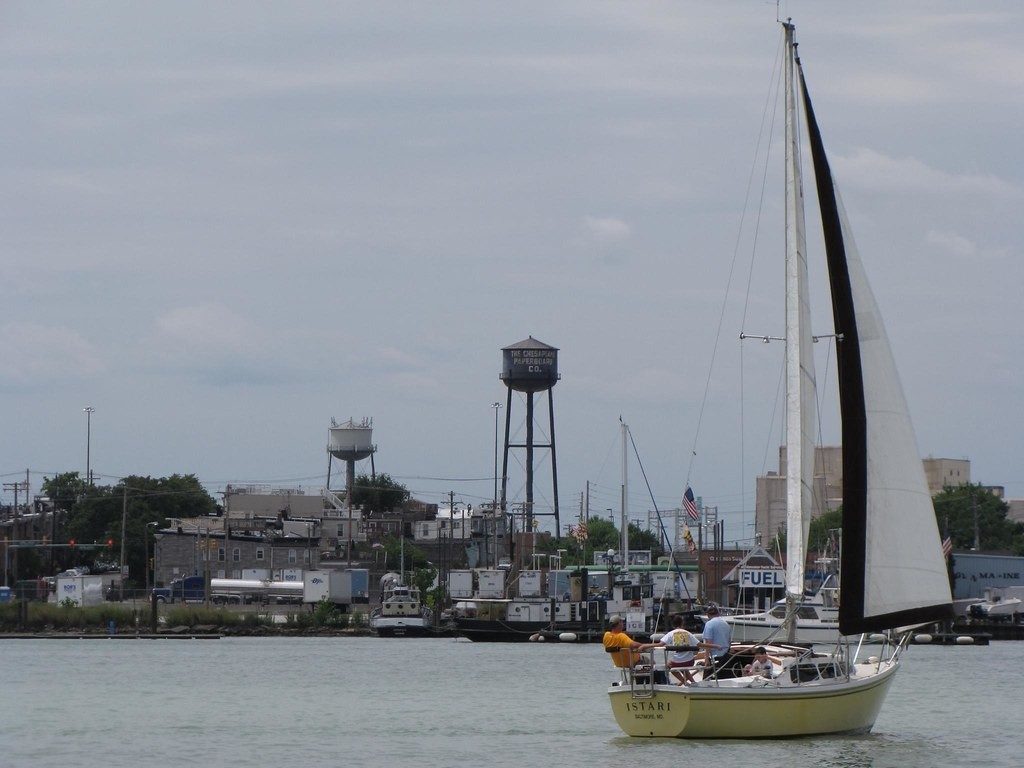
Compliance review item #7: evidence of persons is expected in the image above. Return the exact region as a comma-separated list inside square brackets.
[702, 603, 733, 679]
[603, 615, 655, 668]
[744, 647, 782, 679]
[637, 615, 724, 686]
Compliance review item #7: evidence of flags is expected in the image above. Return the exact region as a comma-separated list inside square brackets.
[683, 521, 698, 556]
[683, 487, 699, 520]
[576, 516, 588, 543]
[942, 524, 953, 555]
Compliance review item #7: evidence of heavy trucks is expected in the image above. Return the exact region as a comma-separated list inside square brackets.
[149, 577, 304, 606]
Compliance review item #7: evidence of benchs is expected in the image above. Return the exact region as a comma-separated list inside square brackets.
[605, 646, 721, 690]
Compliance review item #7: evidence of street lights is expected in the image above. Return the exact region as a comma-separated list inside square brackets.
[82, 407, 96, 484]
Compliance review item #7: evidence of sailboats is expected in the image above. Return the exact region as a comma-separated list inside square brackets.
[609, 0, 954, 738]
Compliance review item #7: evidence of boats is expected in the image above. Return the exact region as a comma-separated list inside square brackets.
[367, 415, 879, 644]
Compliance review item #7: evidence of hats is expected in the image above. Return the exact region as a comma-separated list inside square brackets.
[609, 615, 625, 624]
[704, 605, 718, 613]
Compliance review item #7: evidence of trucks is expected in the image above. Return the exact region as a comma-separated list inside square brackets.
[48, 576, 103, 606]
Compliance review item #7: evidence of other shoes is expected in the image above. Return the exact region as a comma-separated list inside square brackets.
[691, 682, 697, 686]
[685, 683, 691, 687]
